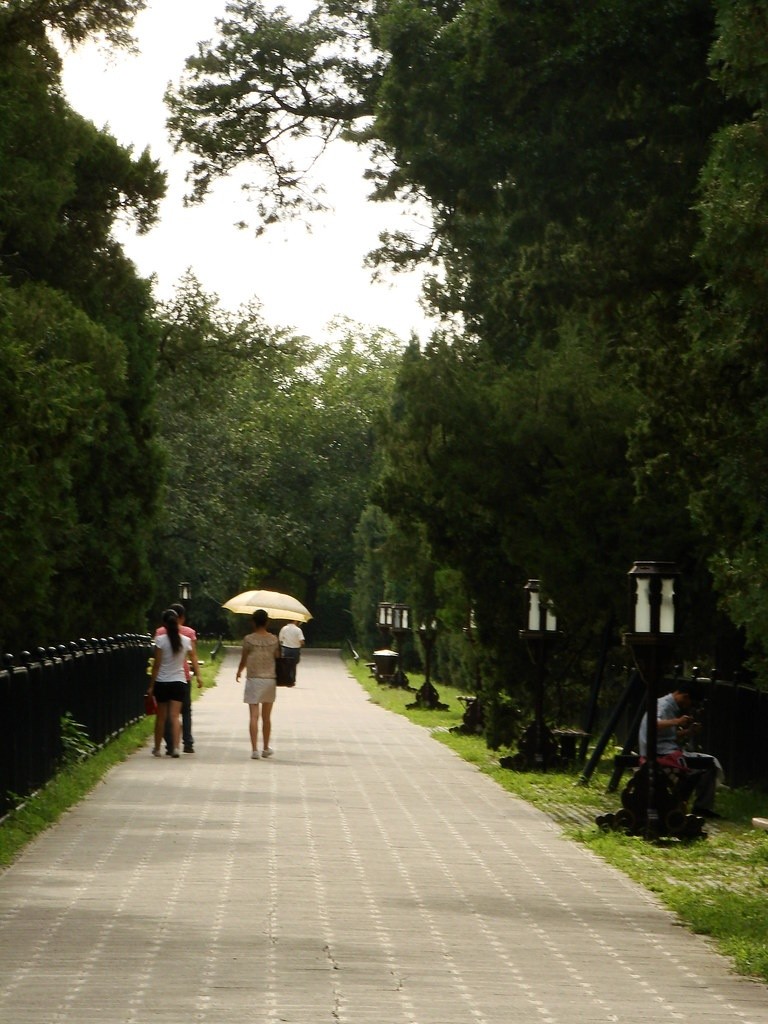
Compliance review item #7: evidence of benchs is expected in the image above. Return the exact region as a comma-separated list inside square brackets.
[456, 696, 477, 711]
[609, 754, 724, 820]
[365, 663, 376, 678]
[518, 729, 592, 761]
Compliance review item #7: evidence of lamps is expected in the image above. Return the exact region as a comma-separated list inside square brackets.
[178, 581, 192, 607]
[595, 558, 710, 849]
[499, 578, 564, 773]
[449, 607, 485, 736]
[389, 603, 413, 687]
[405, 614, 450, 712]
[375, 602, 392, 629]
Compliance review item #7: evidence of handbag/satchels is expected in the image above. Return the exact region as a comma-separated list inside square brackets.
[274, 657, 295, 686]
[144, 694, 159, 716]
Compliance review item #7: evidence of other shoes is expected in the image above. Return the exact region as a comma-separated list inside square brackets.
[184, 746, 195, 753]
[166, 751, 172, 756]
[261, 747, 273, 757]
[691, 808, 721, 819]
[252, 751, 259, 759]
[172, 750, 180, 757]
[152, 751, 161, 757]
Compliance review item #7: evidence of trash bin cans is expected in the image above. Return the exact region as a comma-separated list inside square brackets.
[372, 649, 399, 677]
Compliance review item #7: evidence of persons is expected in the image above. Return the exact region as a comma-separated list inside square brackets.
[236, 610, 280, 759]
[278, 620, 305, 686]
[147, 604, 202, 758]
[640, 682, 722, 819]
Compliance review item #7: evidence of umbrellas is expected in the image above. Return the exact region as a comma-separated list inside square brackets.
[222, 590, 313, 624]
